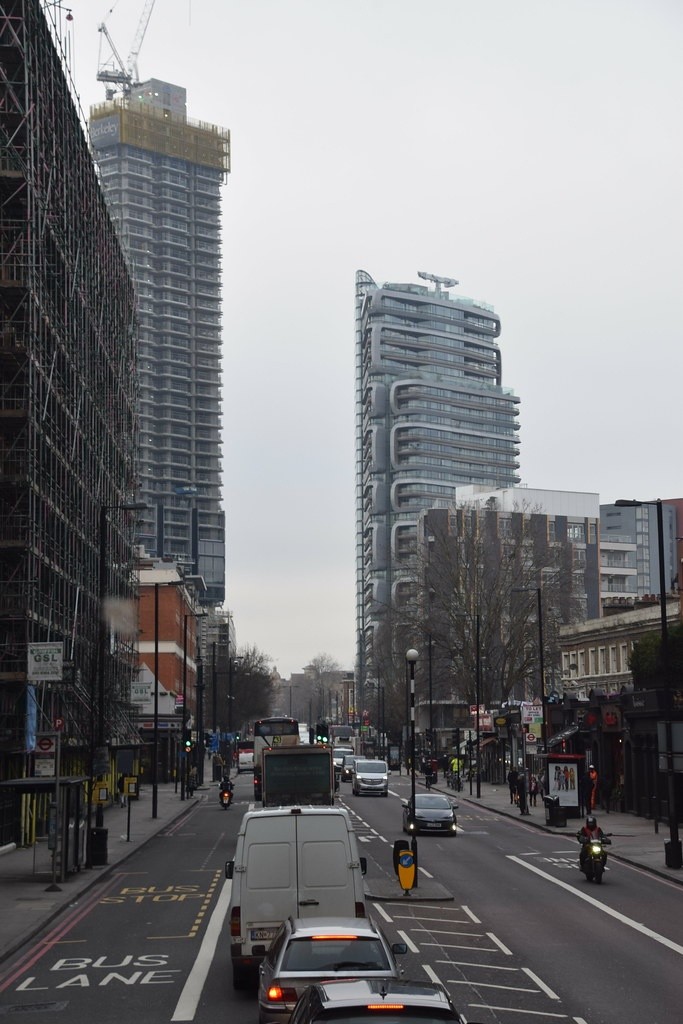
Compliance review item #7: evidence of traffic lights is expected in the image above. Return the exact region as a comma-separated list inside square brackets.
[315, 724, 329, 744]
[183, 738, 192, 753]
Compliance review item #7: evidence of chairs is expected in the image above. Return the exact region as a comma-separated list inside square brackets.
[335, 942, 380, 970]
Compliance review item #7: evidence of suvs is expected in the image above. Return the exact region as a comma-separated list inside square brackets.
[251, 916, 407, 1024]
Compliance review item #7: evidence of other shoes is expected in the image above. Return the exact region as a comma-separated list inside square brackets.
[231, 801, 234, 804]
[219, 800, 222, 803]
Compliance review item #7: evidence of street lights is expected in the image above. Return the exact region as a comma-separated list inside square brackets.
[211, 641, 232, 782]
[180, 610, 209, 801]
[393, 652, 410, 776]
[367, 686, 385, 761]
[510, 585, 550, 795]
[455, 611, 481, 799]
[615, 499, 683, 868]
[228, 655, 244, 760]
[85, 501, 157, 868]
[404, 647, 420, 888]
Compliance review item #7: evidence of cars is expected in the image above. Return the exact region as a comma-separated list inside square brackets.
[286, 976, 469, 1024]
[340, 754, 367, 782]
[401, 793, 458, 837]
[350, 758, 393, 797]
[332, 747, 354, 773]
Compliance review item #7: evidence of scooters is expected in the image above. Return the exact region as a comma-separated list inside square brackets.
[217, 783, 235, 811]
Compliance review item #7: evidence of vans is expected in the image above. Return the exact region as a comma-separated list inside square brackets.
[223, 804, 367, 992]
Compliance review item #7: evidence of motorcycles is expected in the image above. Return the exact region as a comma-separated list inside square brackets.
[576, 830, 613, 884]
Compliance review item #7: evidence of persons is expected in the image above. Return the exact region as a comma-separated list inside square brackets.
[553, 766, 576, 792]
[579, 817, 607, 872]
[441, 754, 463, 778]
[219, 775, 234, 804]
[117, 773, 127, 808]
[424, 763, 432, 790]
[189, 771, 195, 798]
[508, 768, 545, 808]
[581, 764, 611, 815]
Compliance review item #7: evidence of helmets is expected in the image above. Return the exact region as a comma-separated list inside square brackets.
[585, 816, 597, 830]
[223, 775, 229, 781]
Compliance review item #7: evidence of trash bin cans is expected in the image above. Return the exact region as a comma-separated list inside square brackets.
[544, 795, 559, 826]
[90, 827, 109, 864]
[554, 807, 567, 827]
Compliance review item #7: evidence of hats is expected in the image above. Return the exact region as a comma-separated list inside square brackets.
[589, 765, 594, 768]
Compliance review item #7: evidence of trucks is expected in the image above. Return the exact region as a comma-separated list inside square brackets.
[260, 743, 336, 807]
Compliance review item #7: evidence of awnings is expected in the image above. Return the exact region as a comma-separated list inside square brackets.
[479, 737, 496, 752]
[537, 725, 578, 748]
[452, 741, 467, 750]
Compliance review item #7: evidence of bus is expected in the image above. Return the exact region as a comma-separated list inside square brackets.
[237, 740, 255, 774]
[251, 715, 301, 803]
